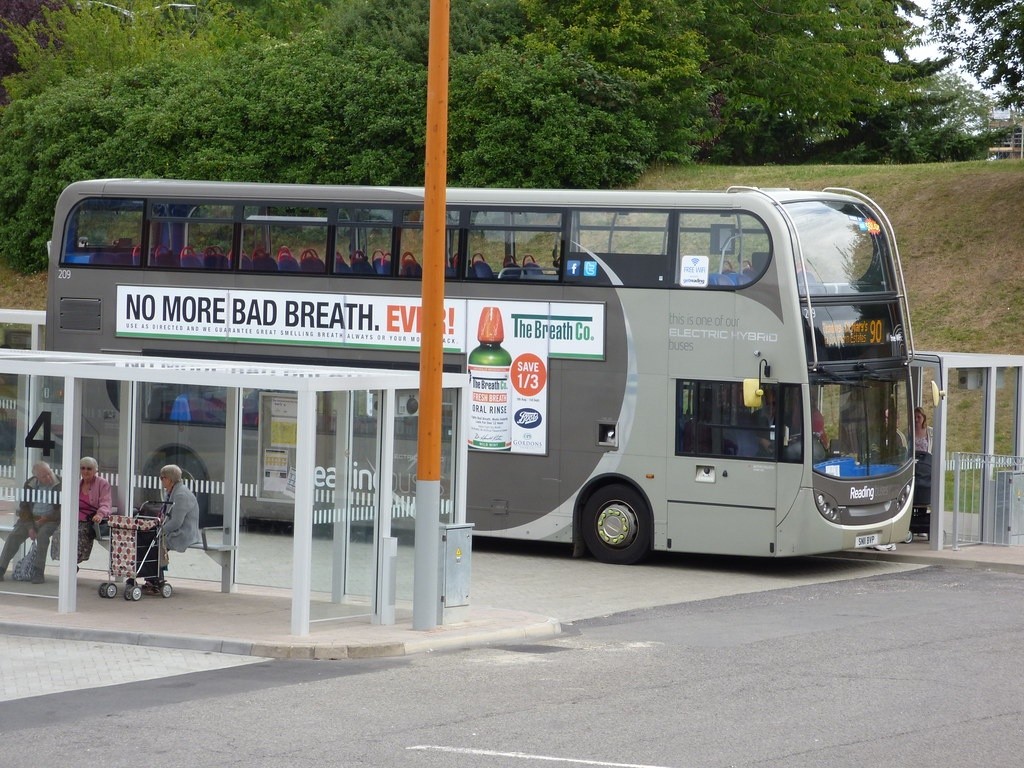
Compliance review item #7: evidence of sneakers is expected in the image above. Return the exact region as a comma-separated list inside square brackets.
[867, 543, 896, 551]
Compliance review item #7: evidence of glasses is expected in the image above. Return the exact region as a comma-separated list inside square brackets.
[161, 476, 166, 480]
[81, 467, 92, 470]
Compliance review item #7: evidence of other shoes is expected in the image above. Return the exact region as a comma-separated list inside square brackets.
[917, 533, 927, 537]
[32, 575, 44, 583]
[0, 572, 3, 580]
[142, 579, 162, 594]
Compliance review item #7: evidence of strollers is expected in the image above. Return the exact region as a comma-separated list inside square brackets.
[903, 451, 932, 544]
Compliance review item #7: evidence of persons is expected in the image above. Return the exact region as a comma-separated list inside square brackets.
[242, 393, 258, 426]
[885, 407, 906, 451]
[907, 407, 934, 457]
[138, 464, 199, 595]
[0, 462, 62, 584]
[748, 390, 791, 453]
[51, 457, 112, 574]
[791, 387, 828, 449]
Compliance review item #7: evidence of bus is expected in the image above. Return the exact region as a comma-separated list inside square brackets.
[40, 178, 948, 567]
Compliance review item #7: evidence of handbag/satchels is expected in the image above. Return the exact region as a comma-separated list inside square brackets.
[13, 538, 38, 580]
[90, 524, 109, 538]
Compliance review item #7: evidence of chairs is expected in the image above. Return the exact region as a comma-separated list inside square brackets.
[91, 244, 544, 281]
[712, 262, 754, 288]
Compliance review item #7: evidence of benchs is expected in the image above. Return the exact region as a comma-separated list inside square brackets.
[0, 527, 237, 592]
